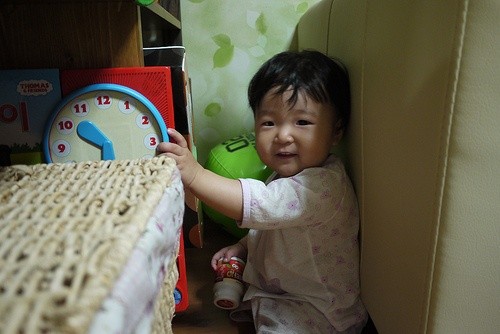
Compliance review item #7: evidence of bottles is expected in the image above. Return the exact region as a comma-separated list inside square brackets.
[214, 257, 245, 309]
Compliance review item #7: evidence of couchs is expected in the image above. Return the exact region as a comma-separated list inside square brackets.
[298, 0, 499, 333]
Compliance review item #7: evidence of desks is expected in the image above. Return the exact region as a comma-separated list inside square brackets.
[0, 155, 186, 334]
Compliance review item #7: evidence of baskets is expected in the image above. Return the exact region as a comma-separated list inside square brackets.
[0, 153, 185, 334]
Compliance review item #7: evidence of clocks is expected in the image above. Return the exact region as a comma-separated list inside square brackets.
[44, 83, 169, 164]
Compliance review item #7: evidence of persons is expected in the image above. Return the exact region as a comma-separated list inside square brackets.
[157, 50, 369, 334]
